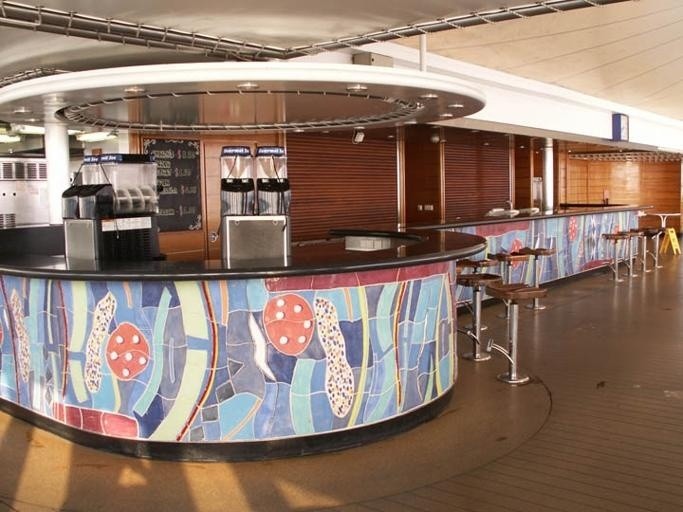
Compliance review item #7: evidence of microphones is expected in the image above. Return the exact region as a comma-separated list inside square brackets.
[456, 247, 555, 386]
[602, 227, 665, 284]
